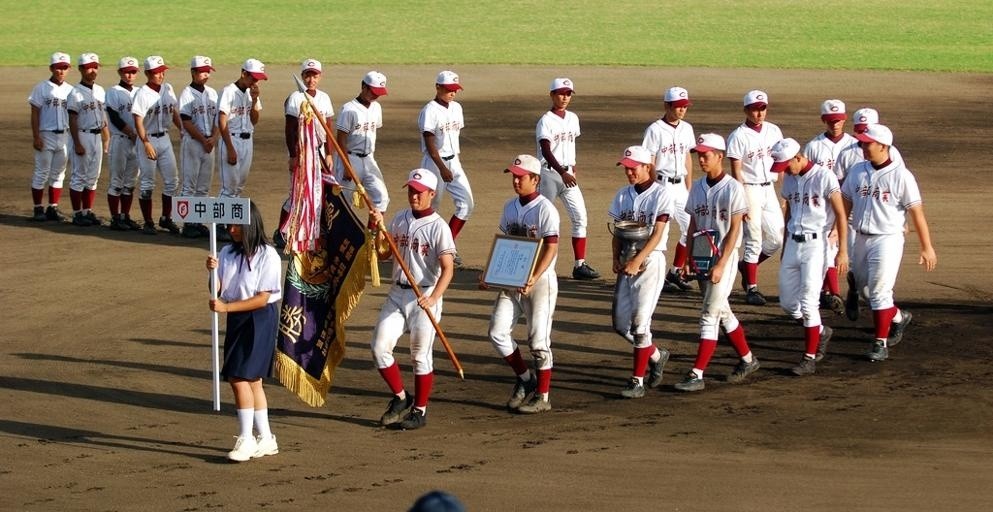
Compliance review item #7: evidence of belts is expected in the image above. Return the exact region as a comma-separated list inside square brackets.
[792, 233, 816, 241]
[657, 175, 680, 183]
[52, 129, 250, 139]
[442, 155, 455, 161]
[560, 166, 568, 171]
[396, 280, 428, 289]
[348, 151, 367, 157]
[747, 182, 770, 186]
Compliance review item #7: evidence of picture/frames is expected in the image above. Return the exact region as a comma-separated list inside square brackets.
[482, 235, 543, 292]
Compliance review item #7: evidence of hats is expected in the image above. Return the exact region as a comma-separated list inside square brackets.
[437, 71, 463, 91]
[690, 133, 726, 153]
[51, 52, 267, 80]
[664, 87, 691, 107]
[301, 59, 321, 73]
[616, 145, 651, 168]
[504, 155, 541, 176]
[364, 71, 387, 96]
[550, 78, 574, 94]
[402, 169, 437, 193]
[743, 90, 892, 173]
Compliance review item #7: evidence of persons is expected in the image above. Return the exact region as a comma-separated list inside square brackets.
[536, 79, 601, 278]
[203, 199, 282, 463]
[641, 86, 697, 290]
[370, 169, 457, 431]
[479, 155, 560, 413]
[608, 144, 675, 399]
[729, 90, 937, 373]
[675, 134, 761, 390]
[283, 59, 389, 209]
[417, 72, 475, 269]
[28, 53, 268, 240]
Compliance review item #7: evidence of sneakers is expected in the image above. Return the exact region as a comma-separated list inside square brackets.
[573, 262, 599, 278]
[738, 261, 767, 305]
[727, 354, 761, 381]
[34, 205, 232, 241]
[508, 373, 552, 412]
[665, 269, 692, 293]
[228, 434, 279, 461]
[620, 348, 705, 398]
[381, 390, 426, 429]
[793, 290, 913, 375]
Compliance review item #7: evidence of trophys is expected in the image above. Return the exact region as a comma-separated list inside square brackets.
[607, 222, 654, 273]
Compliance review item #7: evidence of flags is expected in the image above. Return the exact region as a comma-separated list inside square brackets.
[271, 144, 379, 407]
